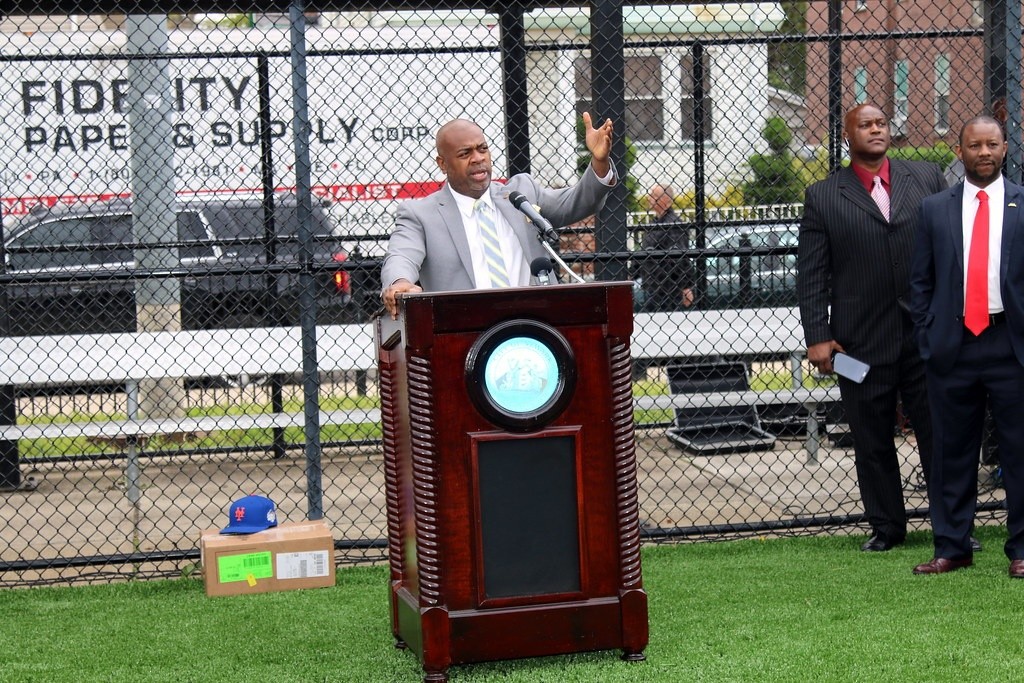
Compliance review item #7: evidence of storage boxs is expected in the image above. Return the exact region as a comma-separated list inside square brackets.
[199, 520, 336, 596]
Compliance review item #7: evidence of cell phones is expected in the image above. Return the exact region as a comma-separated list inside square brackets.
[830, 350, 871, 384]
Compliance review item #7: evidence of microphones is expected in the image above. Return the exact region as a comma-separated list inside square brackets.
[509, 191, 559, 242]
[530, 257, 552, 285]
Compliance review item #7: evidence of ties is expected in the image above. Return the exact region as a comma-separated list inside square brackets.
[871, 176, 890, 222]
[474, 199, 510, 287]
[965, 191, 988, 336]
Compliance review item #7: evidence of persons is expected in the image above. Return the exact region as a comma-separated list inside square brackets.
[798, 104, 983, 552]
[911, 115, 1024, 578]
[380, 111, 620, 321]
[627, 183, 698, 312]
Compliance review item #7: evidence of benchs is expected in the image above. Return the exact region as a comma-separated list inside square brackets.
[0, 307, 842, 466]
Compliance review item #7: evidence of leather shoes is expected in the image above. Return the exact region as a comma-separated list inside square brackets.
[861, 531, 906, 551]
[912, 557, 973, 574]
[1008, 559, 1024, 577]
[969, 534, 981, 551]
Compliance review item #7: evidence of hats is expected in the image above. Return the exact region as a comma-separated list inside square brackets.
[221, 495, 277, 534]
